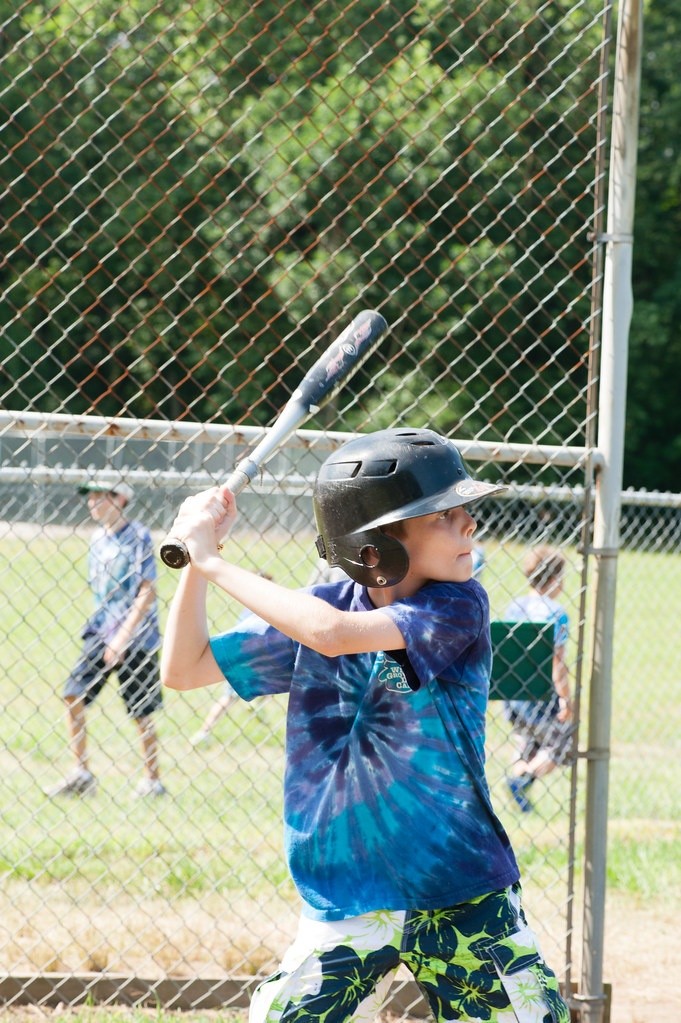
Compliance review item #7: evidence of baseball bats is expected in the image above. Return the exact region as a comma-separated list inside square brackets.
[162, 311, 385, 566]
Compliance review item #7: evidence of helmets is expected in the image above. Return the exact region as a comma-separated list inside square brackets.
[314, 426, 507, 589]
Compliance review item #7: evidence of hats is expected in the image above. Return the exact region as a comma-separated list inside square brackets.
[74, 470, 136, 500]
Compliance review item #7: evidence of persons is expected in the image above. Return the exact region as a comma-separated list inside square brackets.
[160, 427, 570, 1023]
[501, 549, 577, 814]
[46, 473, 165, 801]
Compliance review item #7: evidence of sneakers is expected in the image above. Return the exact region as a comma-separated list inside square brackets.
[42, 768, 97, 797]
[126, 778, 164, 798]
[507, 772, 533, 812]
